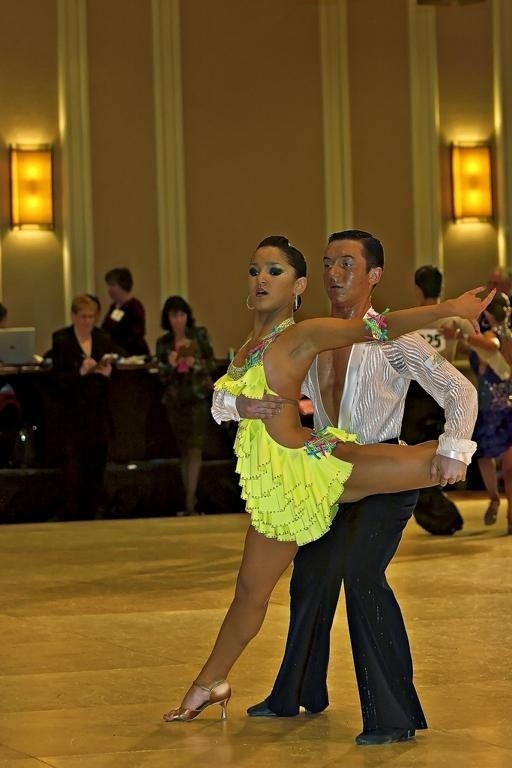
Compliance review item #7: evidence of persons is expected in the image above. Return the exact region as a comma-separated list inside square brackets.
[156, 231, 499, 725]
[236, 228, 484, 745]
[398, 264, 512, 535]
[0, 267, 220, 516]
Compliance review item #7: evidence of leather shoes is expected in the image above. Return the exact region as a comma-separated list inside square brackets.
[355, 726, 416, 745]
[245, 695, 301, 718]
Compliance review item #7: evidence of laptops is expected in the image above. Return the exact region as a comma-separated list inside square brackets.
[0, 327, 34, 366]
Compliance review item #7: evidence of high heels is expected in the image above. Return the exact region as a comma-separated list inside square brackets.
[483, 496, 500, 526]
[164, 679, 232, 722]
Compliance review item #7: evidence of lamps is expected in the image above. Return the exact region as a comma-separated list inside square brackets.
[7, 143, 57, 233]
[445, 140, 499, 227]
